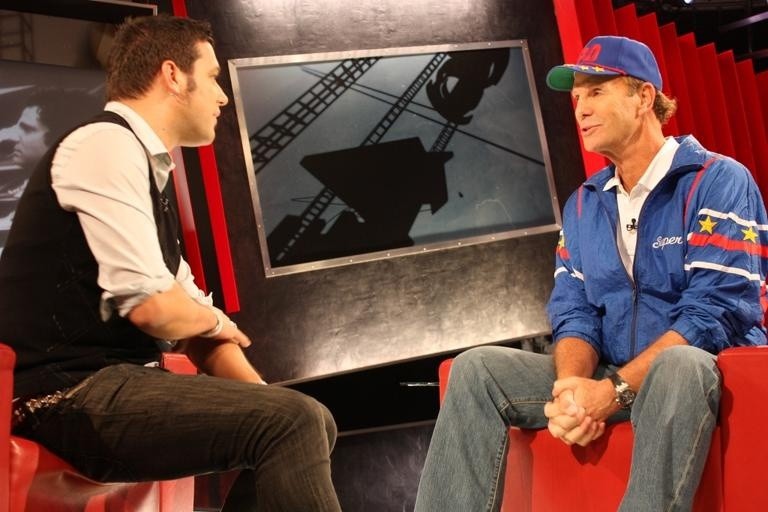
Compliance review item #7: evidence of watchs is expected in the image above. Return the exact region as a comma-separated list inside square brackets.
[607, 371, 637, 413]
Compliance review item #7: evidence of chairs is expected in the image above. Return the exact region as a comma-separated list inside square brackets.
[436, 344, 768, 512]
[0, 343, 199, 512]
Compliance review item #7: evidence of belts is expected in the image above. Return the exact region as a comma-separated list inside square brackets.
[10, 388, 71, 431]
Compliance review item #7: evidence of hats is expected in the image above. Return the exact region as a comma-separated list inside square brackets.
[547, 36, 662, 92]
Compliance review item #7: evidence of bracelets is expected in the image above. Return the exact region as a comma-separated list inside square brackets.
[199, 310, 223, 339]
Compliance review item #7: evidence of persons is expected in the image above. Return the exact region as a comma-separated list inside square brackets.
[0, 15, 342, 511]
[0, 84, 97, 258]
[414, 35, 768, 512]
